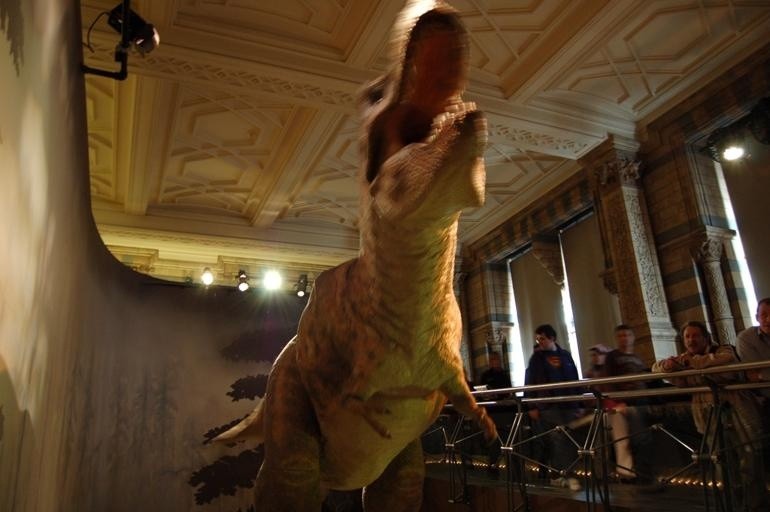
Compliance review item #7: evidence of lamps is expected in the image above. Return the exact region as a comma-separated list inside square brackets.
[84, 2, 161, 81]
[293, 273, 308, 299]
[236, 270, 251, 292]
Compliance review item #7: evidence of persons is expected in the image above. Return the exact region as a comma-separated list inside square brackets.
[734, 297, 770, 408]
[605, 324, 669, 496]
[532, 343, 542, 354]
[650, 319, 770, 511]
[584, 343, 617, 477]
[524, 323, 584, 491]
[479, 351, 513, 482]
